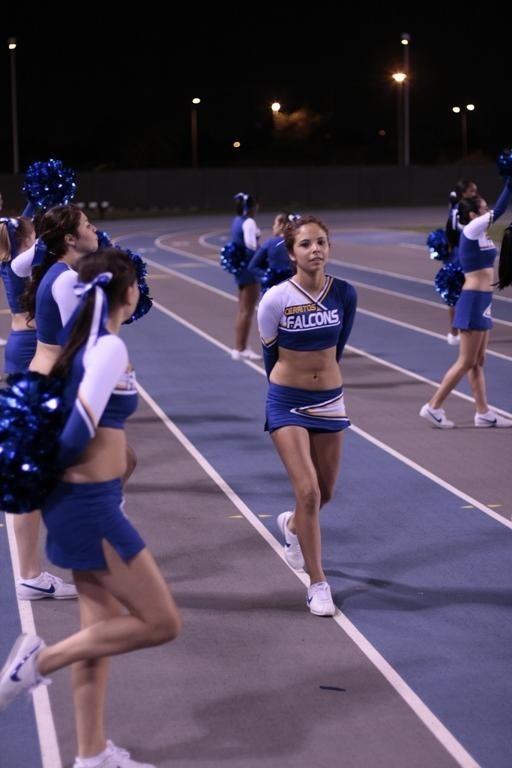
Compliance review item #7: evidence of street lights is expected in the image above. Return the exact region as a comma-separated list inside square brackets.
[190, 98, 201, 169]
[453, 102, 474, 158]
[272, 103, 280, 165]
[8, 42, 21, 216]
[392, 33, 409, 168]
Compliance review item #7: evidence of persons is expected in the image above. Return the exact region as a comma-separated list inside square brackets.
[232, 193, 262, 360]
[18, 203, 101, 602]
[258, 216, 358, 617]
[418, 155, 512, 430]
[247, 212, 296, 296]
[445, 181, 478, 346]
[1, 249, 180, 767]
[1, 214, 45, 378]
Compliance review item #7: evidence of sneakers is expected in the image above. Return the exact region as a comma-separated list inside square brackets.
[232, 349, 263, 361]
[17, 571, 79, 600]
[0, 631, 46, 712]
[277, 511, 305, 569]
[448, 332, 460, 345]
[307, 581, 335, 616]
[419, 403, 454, 429]
[72, 739, 154, 768]
[475, 410, 512, 428]
